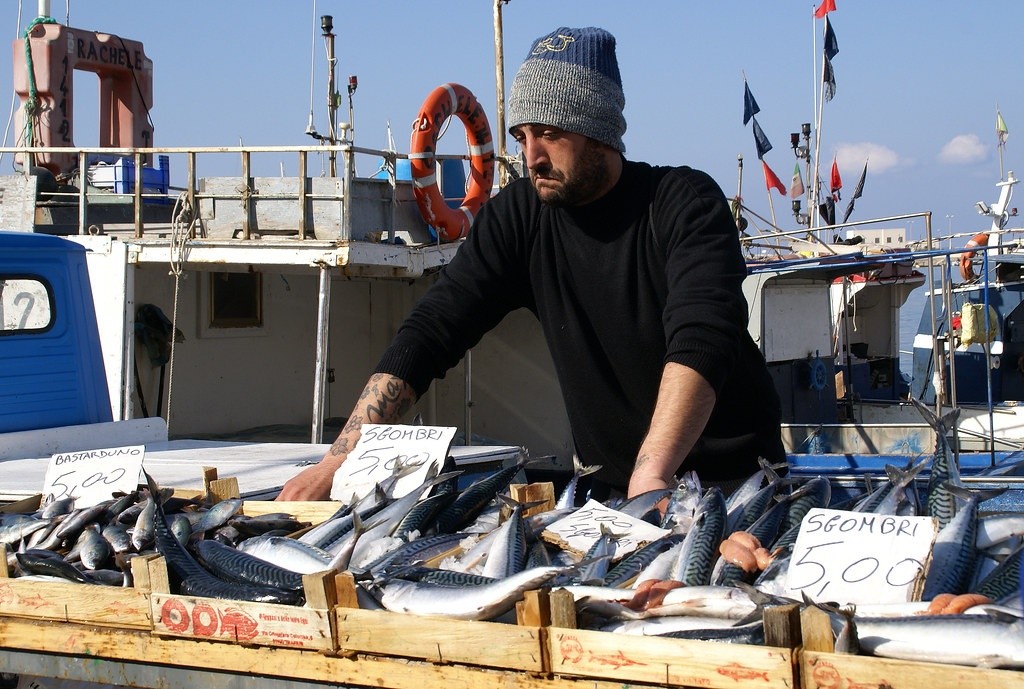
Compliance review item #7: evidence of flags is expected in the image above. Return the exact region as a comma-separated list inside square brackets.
[742, 0, 869, 229]
[996, 111, 1009, 147]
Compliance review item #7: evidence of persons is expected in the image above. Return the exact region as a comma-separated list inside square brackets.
[272, 26, 785, 523]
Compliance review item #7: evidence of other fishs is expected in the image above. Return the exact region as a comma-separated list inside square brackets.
[0, 446, 1024, 672]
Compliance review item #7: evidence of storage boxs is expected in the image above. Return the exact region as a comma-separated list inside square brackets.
[0, 468, 1024, 689]
[88, 156, 170, 195]
[908, 333, 1003, 406]
[198, 178, 434, 249]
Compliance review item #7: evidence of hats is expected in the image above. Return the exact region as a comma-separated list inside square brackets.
[507, 26, 628, 153]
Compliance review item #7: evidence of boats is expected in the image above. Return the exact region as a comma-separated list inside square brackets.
[910, 100, 1024, 405]
[723, 1, 926, 403]
[1, 0, 516, 442]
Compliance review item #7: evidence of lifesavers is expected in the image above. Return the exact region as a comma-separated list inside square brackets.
[960, 233, 1003, 283]
[408, 79, 496, 242]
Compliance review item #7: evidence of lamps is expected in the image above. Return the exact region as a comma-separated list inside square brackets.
[791, 200, 810, 226]
[790, 133, 807, 158]
[801, 123, 811, 139]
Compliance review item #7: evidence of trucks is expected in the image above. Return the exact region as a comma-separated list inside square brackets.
[1, 231, 1024, 689]
